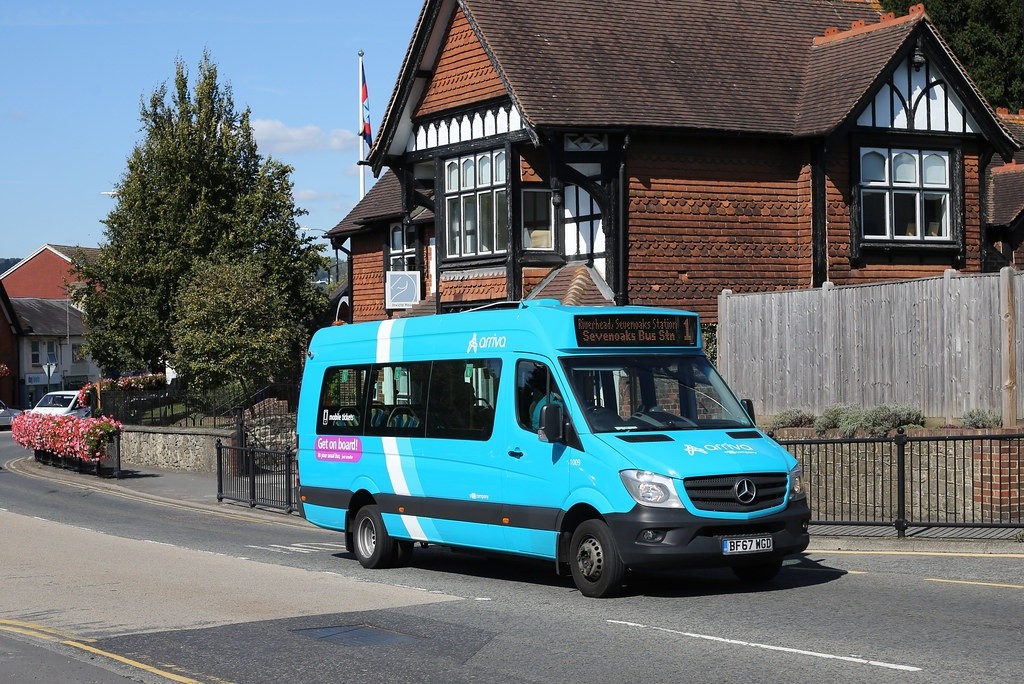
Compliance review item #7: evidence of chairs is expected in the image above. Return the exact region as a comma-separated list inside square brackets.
[386, 407, 421, 428]
[370, 401, 390, 428]
[528, 378, 547, 423]
[332, 407, 361, 426]
[464, 396, 494, 430]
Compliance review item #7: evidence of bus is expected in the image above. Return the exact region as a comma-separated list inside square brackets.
[295, 299, 813, 598]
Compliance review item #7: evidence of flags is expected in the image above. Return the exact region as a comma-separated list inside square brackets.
[362, 62, 372, 149]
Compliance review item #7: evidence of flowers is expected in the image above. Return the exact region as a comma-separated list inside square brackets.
[77, 371, 168, 408]
[11, 411, 126, 463]
[0, 363, 10, 376]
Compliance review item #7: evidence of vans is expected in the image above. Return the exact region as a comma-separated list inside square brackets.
[30, 389, 96, 419]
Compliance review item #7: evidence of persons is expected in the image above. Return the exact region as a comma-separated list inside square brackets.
[533, 367, 580, 432]
[451, 382, 476, 428]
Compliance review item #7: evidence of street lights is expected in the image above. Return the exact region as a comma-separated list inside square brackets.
[300, 225, 339, 283]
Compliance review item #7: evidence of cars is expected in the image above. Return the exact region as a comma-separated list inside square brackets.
[0, 400, 24, 426]
